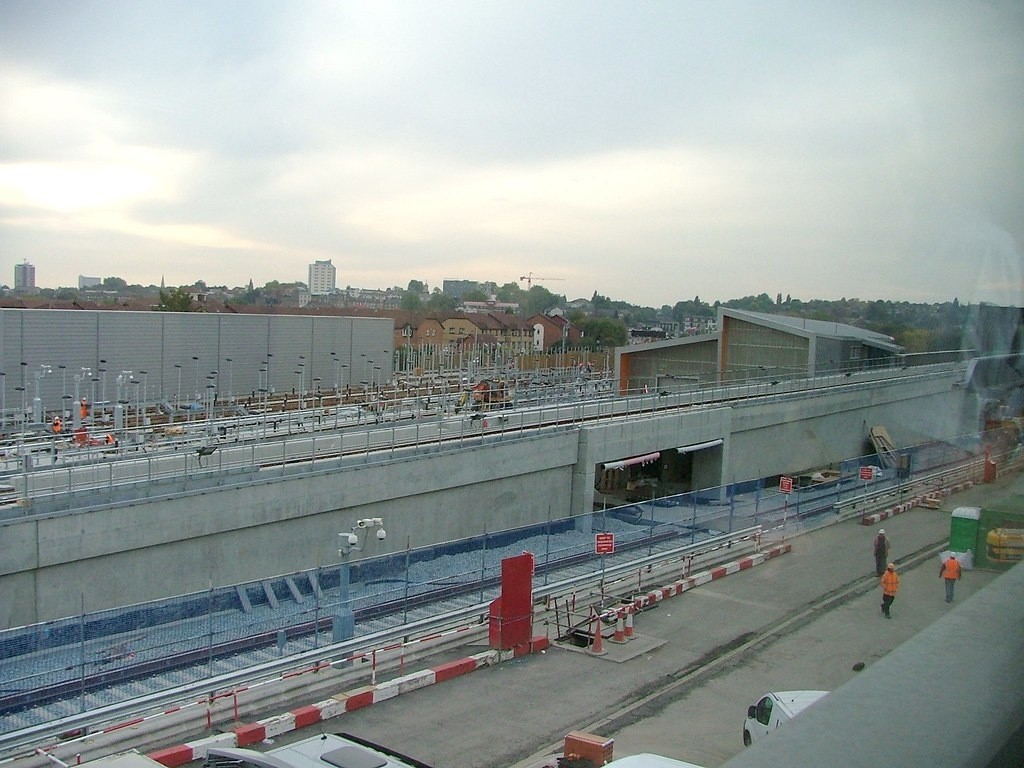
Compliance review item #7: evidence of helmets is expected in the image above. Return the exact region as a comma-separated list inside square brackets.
[879, 529, 885, 533]
[950, 552, 956, 557]
[887, 563, 895, 569]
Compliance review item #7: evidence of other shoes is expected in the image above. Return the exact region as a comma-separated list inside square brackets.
[881, 604, 884, 613]
[884, 613, 890, 618]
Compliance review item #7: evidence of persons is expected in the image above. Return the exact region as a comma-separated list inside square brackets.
[939, 552, 961, 603]
[873, 529, 891, 577]
[52, 417, 64, 433]
[81, 397, 87, 418]
[881, 563, 900, 619]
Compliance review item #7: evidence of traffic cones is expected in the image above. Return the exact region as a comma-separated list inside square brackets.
[608, 609, 630, 643]
[585, 619, 610, 651]
[623, 605, 639, 640]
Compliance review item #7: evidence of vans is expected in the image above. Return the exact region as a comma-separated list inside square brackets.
[742, 690, 831, 748]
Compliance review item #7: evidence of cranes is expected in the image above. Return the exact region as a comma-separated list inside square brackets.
[519, 271, 565, 290]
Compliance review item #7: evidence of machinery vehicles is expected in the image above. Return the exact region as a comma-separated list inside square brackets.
[455, 377, 515, 420]
